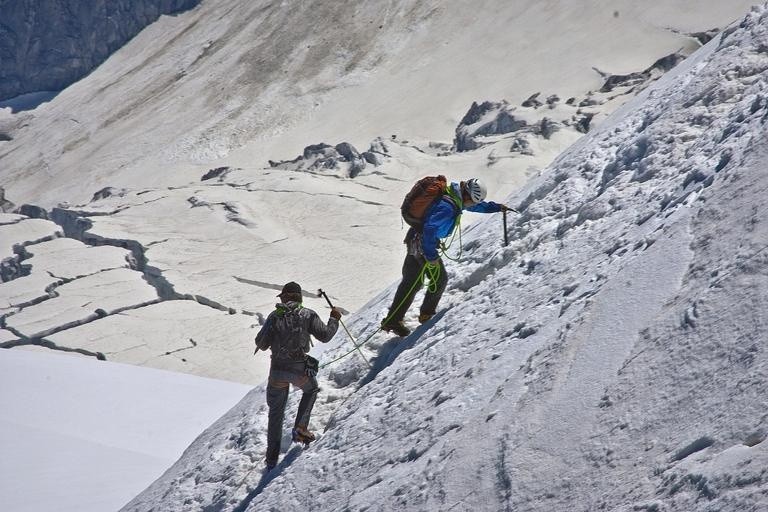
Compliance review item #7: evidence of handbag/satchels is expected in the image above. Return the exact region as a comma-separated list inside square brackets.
[305, 355, 319, 377]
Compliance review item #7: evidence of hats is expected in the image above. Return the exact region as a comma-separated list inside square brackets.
[276, 281, 301, 297]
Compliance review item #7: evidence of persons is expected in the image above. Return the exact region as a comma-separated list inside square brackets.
[253, 280, 349, 467]
[381, 175, 518, 337]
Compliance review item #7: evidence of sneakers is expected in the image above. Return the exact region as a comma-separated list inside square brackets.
[418, 312, 432, 324]
[292, 428, 315, 443]
[265, 459, 277, 470]
[381, 318, 410, 336]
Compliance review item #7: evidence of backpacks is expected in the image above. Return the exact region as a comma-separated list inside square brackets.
[400, 175, 463, 231]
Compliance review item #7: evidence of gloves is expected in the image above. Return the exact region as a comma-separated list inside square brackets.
[329, 308, 342, 320]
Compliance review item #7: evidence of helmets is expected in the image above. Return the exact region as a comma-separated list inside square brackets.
[464, 178, 488, 204]
